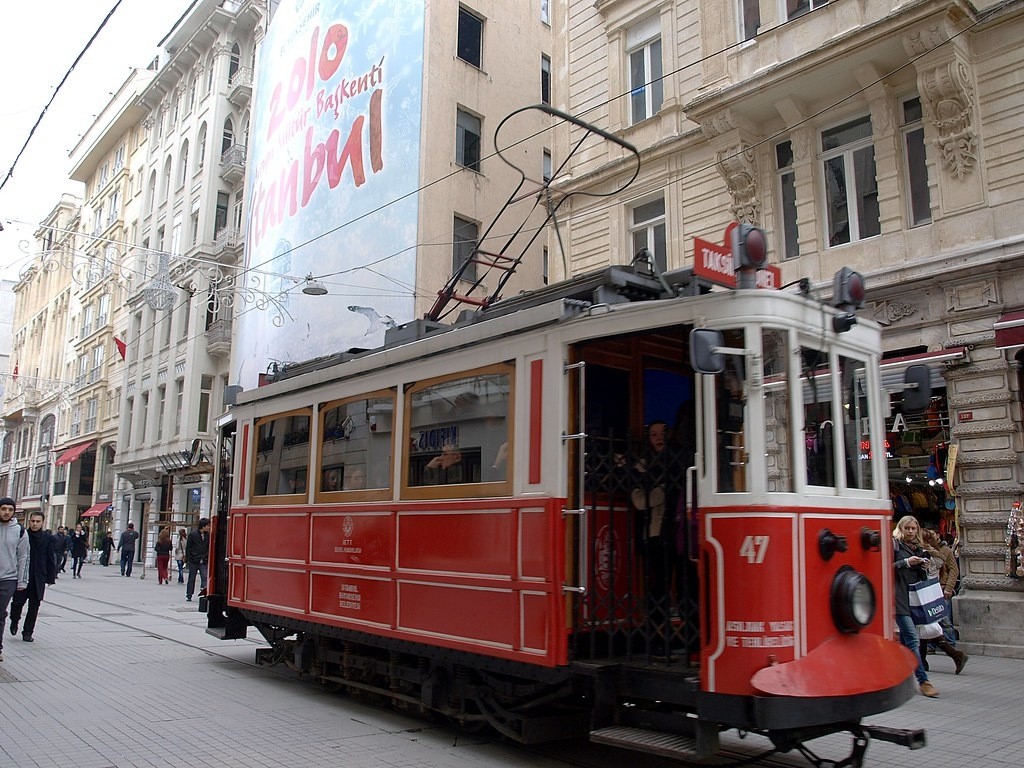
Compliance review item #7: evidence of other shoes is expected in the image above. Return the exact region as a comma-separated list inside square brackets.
[186, 594, 192, 601]
[919, 680, 939, 697]
[0, 654, 3, 661]
[920, 658, 929, 671]
[689, 652, 700, 667]
[165, 578, 168, 584]
[648, 652, 667, 664]
[952, 651, 968, 675]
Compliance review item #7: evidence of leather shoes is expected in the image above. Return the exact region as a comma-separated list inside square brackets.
[10, 621, 18, 635]
[22, 635, 34, 642]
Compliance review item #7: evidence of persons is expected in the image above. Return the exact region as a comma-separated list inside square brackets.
[117, 523, 138, 577]
[423, 437, 465, 486]
[0, 498, 31, 661]
[633, 400, 701, 666]
[10, 512, 55, 641]
[174, 529, 188, 585]
[324, 469, 366, 491]
[186, 518, 211, 602]
[155, 529, 173, 585]
[47, 523, 89, 579]
[102, 531, 116, 567]
[893, 516, 970, 697]
[483, 441, 509, 482]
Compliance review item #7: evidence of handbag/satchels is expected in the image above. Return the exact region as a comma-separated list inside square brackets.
[909, 566, 949, 625]
[183, 555, 186, 562]
[890, 483, 940, 513]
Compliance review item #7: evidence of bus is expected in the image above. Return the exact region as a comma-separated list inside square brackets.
[191, 100, 930, 768]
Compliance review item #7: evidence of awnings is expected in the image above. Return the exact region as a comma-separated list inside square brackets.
[81, 503, 112, 517]
[56, 441, 95, 466]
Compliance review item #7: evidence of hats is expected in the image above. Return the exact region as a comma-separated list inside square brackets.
[0, 498, 16, 514]
